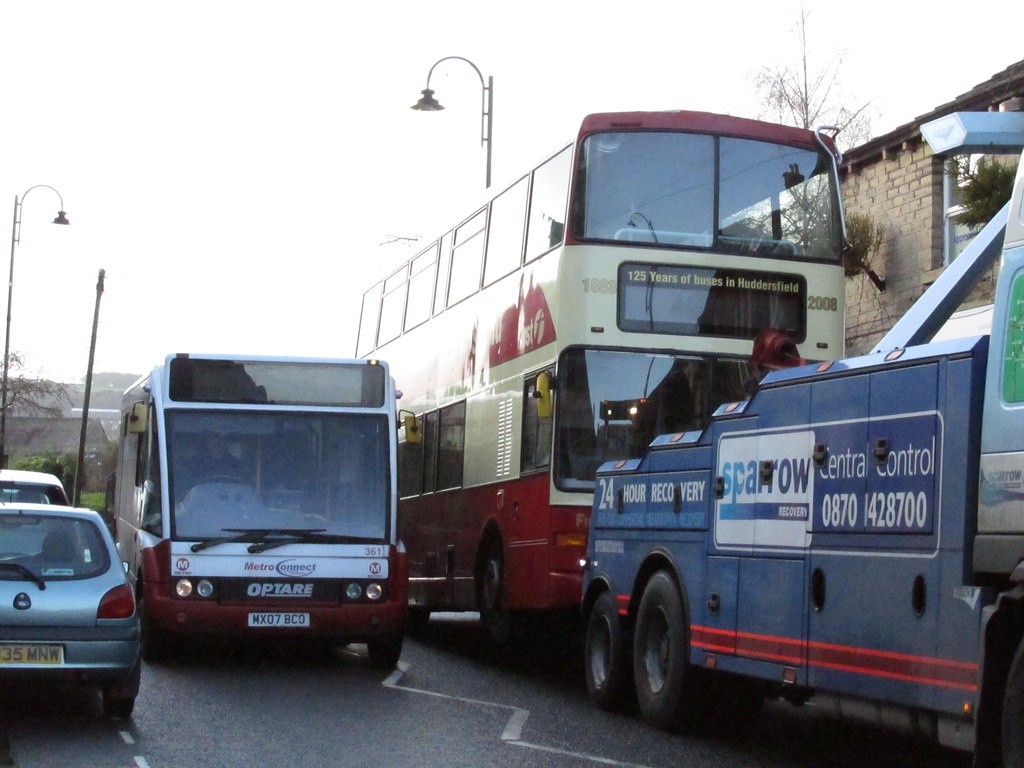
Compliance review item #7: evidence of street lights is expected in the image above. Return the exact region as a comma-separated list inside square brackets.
[0, 183, 70, 470]
[411, 53, 493, 187]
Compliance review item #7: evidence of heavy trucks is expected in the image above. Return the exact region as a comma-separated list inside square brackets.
[581, 107, 1023, 765]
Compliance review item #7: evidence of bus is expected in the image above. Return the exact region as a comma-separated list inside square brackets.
[354, 109, 851, 667]
[117, 352, 407, 668]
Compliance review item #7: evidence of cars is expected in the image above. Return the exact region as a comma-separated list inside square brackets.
[1, 498, 142, 721]
[1, 467, 91, 563]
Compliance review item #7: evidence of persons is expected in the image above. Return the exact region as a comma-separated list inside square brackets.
[179, 427, 252, 508]
